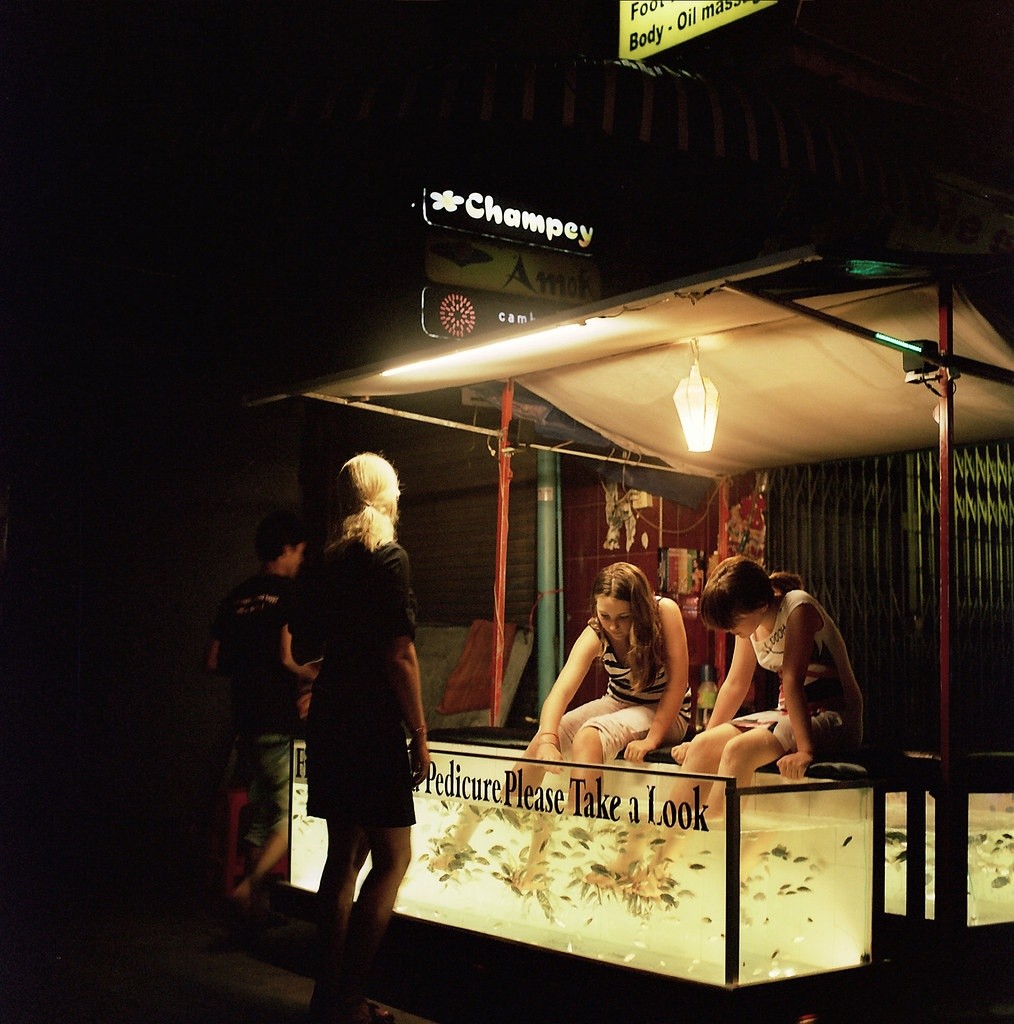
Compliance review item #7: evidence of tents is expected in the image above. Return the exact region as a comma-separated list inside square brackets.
[239, 232, 1012, 768]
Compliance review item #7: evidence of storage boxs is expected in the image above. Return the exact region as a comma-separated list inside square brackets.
[885, 749, 1014, 1011]
[287, 728, 885, 1024]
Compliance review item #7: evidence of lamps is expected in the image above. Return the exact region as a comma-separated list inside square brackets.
[674, 337, 721, 451]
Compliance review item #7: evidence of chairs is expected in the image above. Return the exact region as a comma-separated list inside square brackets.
[414, 622, 538, 730]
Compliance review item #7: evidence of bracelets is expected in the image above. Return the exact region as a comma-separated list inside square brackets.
[535, 743, 561, 751]
[408, 725, 426, 737]
[537, 732, 560, 742]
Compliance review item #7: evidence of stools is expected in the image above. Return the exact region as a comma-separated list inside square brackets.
[208, 783, 288, 902]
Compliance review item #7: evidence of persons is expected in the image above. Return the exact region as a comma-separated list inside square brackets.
[201, 511, 316, 924]
[498, 561, 690, 830]
[690, 558, 703, 594]
[291, 452, 430, 1024]
[584, 556, 861, 893]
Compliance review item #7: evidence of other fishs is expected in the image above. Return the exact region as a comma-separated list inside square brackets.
[292, 785, 1014, 967]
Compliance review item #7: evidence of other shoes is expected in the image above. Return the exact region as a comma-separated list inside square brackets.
[223, 892, 290, 930]
[308, 976, 341, 1023]
[321, 989, 397, 1023]
